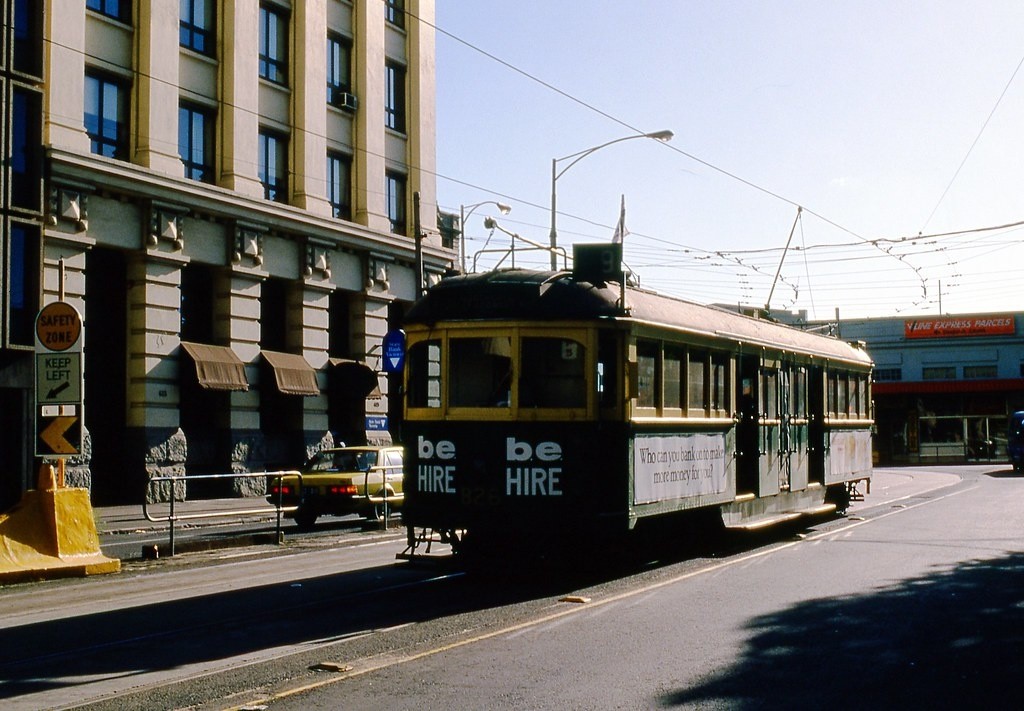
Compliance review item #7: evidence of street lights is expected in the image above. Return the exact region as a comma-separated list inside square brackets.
[461, 201, 511, 275]
[551, 130, 674, 271]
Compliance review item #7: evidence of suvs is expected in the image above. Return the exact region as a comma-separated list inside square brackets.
[1006, 411, 1024, 471]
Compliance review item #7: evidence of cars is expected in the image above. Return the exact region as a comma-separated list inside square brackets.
[936, 424, 994, 457]
[266, 445, 404, 523]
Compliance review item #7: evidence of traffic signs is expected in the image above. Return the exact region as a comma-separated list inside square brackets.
[36, 352, 82, 404]
[35, 353, 81, 403]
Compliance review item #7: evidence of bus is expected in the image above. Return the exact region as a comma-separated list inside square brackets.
[395, 242, 875, 564]
